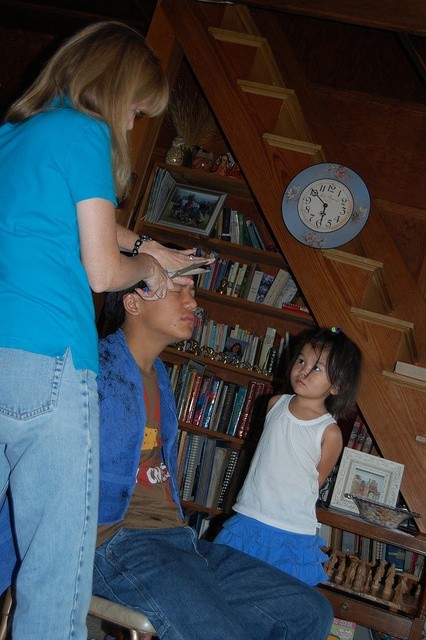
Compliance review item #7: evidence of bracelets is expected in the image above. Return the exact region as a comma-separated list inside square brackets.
[131, 234, 152, 256]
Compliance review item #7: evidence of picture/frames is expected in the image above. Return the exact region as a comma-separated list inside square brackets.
[328, 445, 406, 521]
[156, 182, 228, 237]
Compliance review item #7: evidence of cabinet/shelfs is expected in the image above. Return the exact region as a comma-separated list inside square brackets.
[125, 5, 424, 635]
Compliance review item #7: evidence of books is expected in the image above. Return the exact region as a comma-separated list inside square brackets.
[347, 411, 381, 457]
[318, 520, 424, 578]
[182, 507, 214, 539]
[177, 427, 240, 510]
[143, 165, 268, 250]
[167, 306, 293, 374]
[192, 247, 312, 317]
[163, 357, 274, 439]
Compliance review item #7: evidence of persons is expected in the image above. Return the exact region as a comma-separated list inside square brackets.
[0, 21, 218, 638]
[1, 243, 335, 639]
[210, 327, 362, 589]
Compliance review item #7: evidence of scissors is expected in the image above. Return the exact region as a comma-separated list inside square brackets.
[138, 261, 211, 292]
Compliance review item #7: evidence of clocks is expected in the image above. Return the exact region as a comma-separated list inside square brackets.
[280, 162, 371, 249]
